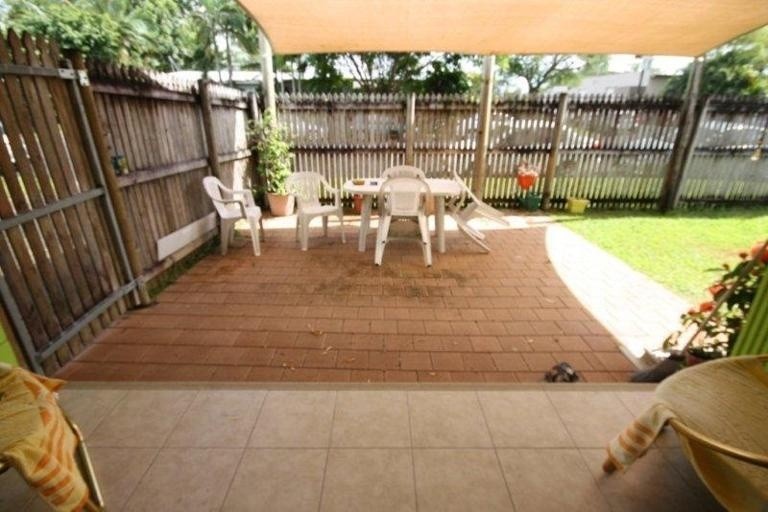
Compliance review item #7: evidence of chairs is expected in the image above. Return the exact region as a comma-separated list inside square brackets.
[202, 175, 268, 258]
[379, 165, 428, 181]
[445, 166, 510, 256]
[368, 173, 435, 269]
[283, 171, 347, 251]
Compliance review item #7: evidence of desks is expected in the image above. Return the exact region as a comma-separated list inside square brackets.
[343, 179, 462, 256]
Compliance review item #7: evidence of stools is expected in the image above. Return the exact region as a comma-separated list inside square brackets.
[0, 361, 107, 512]
[598, 346, 767, 511]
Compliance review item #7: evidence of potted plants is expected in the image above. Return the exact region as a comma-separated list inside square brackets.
[242, 105, 297, 218]
